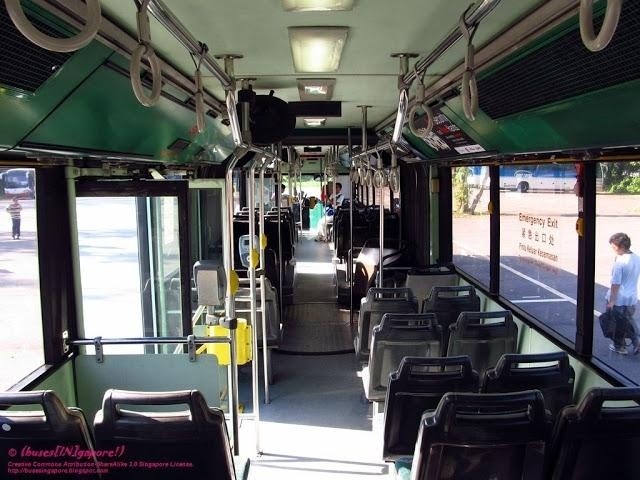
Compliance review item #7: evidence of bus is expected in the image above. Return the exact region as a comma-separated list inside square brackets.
[0, 167, 36, 201]
[465, 160, 609, 194]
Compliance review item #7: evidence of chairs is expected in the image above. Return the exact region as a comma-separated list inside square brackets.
[333, 198, 639, 479]
[1, 206, 296, 479]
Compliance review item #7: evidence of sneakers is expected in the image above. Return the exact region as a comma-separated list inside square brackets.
[633, 336, 640, 355]
[609, 343, 628, 355]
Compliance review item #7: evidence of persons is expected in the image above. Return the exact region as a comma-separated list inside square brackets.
[599, 231, 640, 356]
[314, 182, 345, 243]
[270, 184, 286, 201]
[6, 196, 23, 240]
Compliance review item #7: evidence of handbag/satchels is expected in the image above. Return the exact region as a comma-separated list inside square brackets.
[599, 308, 634, 339]
